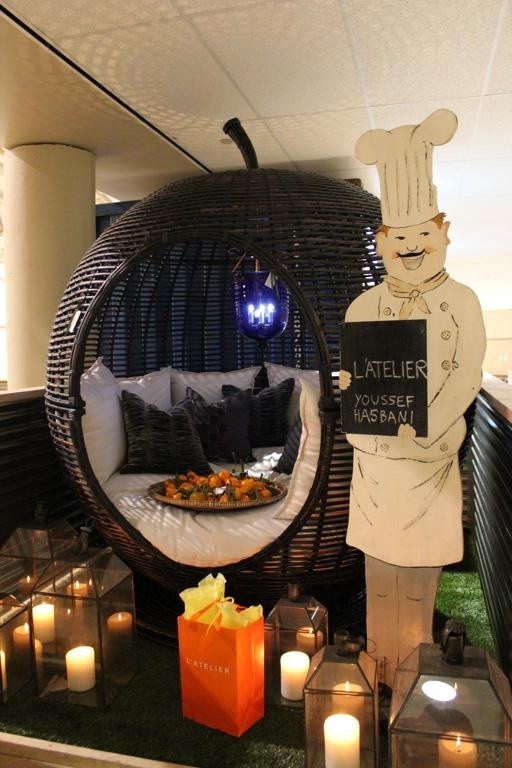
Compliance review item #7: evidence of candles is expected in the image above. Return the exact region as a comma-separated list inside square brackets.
[437, 730, 479, 768]
[275, 626, 365, 768]
[0, 571, 134, 691]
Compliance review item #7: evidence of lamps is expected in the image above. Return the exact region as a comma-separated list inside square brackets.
[232, 255, 290, 347]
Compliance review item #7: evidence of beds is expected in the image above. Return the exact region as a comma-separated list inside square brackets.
[100, 445, 354, 572]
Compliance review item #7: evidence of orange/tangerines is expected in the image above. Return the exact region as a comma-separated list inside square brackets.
[165, 470, 271, 501]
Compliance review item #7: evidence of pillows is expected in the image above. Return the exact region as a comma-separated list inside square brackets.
[160, 366, 260, 406]
[220, 378, 295, 449]
[79, 357, 127, 483]
[273, 411, 301, 475]
[186, 385, 259, 463]
[120, 390, 214, 475]
[116, 366, 172, 427]
[264, 361, 322, 432]
[272, 379, 322, 519]
